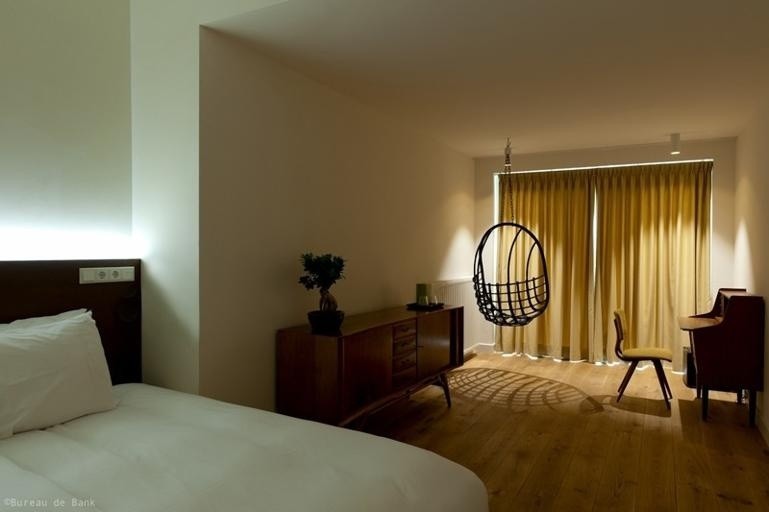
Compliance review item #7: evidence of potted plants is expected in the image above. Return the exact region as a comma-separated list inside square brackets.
[299, 249, 346, 333]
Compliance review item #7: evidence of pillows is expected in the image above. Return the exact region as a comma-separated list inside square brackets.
[1, 308, 118, 441]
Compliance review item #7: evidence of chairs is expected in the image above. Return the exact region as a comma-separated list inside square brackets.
[473, 133, 550, 327]
[613, 306, 674, 411]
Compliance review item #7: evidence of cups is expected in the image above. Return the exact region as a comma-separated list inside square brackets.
[416, 283, 428, 306]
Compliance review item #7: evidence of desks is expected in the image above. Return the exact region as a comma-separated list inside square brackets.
[677, 288, 764, 426]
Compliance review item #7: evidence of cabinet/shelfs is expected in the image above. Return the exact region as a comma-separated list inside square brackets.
[273, 304, 466, 427]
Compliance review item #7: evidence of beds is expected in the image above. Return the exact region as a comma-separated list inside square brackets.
[1, 259, 492, 511]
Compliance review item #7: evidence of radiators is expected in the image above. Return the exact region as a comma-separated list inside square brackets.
[434, 278, 478, 358]
